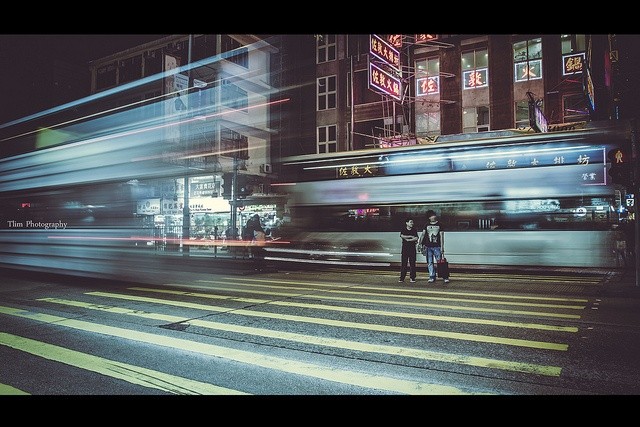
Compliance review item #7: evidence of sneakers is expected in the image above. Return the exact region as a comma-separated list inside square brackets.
[444, 279, 449, 282]
[398, 279, 404, 283]
[410, 278, 415, 283]
[428, 278, 434, 282]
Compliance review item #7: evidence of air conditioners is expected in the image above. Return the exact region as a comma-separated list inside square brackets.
[263, 163, 273, 174]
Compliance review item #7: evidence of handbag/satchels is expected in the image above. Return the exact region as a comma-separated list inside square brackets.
[438, 252, 449, 277]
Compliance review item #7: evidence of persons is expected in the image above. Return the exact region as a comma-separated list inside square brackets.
[418, 210, 450, 283]
[616, 231, 628, 266]
[397, 216, 419, 283]
[241, 214, 266, 260]
